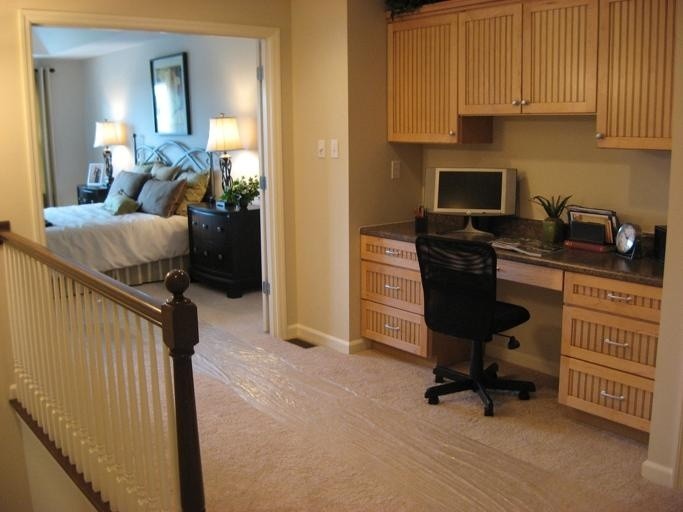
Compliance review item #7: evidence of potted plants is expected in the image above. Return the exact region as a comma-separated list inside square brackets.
[228, 175, 261, 209]
[528, 192, 577, 245]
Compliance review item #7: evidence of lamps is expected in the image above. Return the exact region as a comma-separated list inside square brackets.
[93, 120, 129, 186]
[206, 116, 243, 211]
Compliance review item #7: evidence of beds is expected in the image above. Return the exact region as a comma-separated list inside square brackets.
[43, 133, 213, 300]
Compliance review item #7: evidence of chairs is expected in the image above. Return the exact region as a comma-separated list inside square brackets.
[415, 233, 537, 418]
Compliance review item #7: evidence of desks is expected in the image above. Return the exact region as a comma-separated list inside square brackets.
[360, 210, 664, 434]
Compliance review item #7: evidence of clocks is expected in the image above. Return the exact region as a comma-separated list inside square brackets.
[614, 222, 642, 264]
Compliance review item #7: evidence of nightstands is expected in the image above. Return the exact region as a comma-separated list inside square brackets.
[77, 184, 108, 205]
[187, 201, 263, 299]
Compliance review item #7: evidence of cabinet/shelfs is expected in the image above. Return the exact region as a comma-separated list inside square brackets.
[386, 13, 459, 145]
[459, 0, 598, 116]
[595, 0, 675, 152]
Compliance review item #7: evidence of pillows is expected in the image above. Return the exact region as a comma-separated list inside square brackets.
[136, 177, 188, 218]
[150, 163, 180, 180]
[102, 190, 140, 216]
[175, 170, 209, 216]
[107, 170, 148, 201]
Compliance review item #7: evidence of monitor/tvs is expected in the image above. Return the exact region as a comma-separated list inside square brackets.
[433, 167, 517, 235]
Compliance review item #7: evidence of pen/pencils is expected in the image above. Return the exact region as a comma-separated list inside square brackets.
[414, 205, 428, 218]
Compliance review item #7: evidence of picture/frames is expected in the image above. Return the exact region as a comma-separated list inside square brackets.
[86, 162, 104, 186]
[150, 51, 192, 137]
